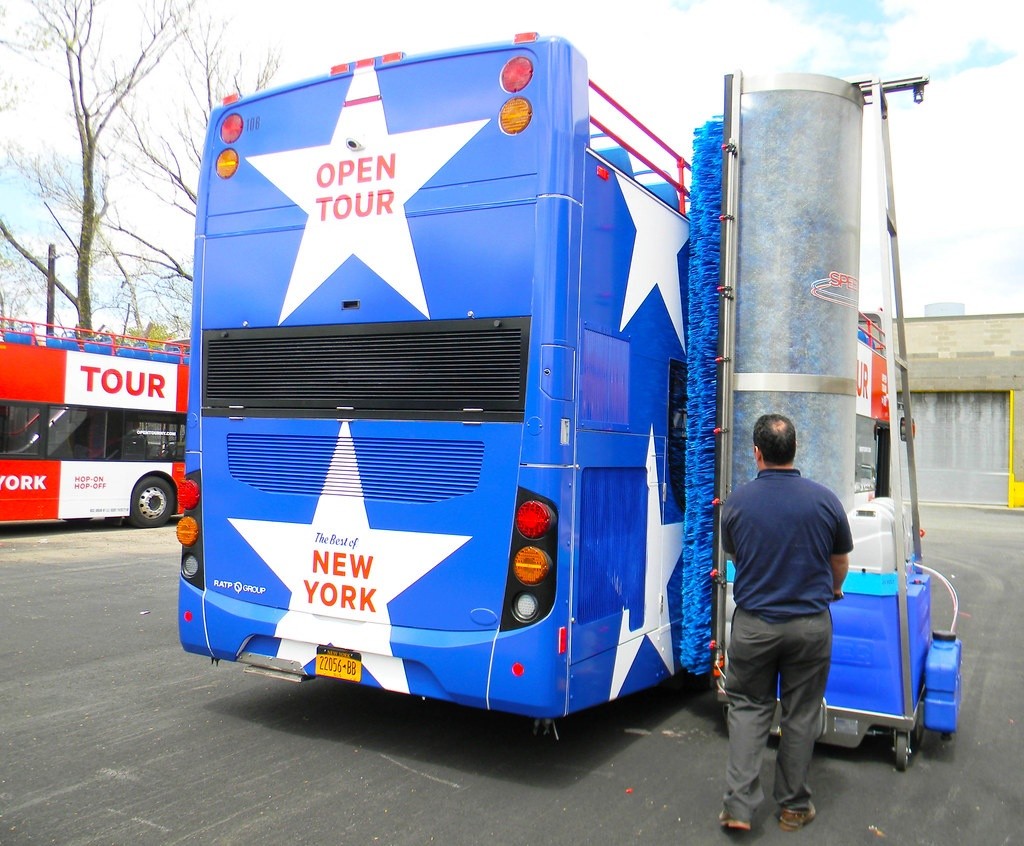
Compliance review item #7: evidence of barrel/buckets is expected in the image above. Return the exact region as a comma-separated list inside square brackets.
[922, 629, 963, 732]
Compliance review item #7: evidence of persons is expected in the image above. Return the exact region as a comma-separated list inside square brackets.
[124, 419, 149, 459]
[720, 414, 855, 833]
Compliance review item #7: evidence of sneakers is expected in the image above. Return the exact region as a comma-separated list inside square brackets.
[717, 809, 751, 831]
[782, 797, 817, 830]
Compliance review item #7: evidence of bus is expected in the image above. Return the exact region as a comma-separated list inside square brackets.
[167, 30, 917, 719]
[0, 317, 190, 529]
